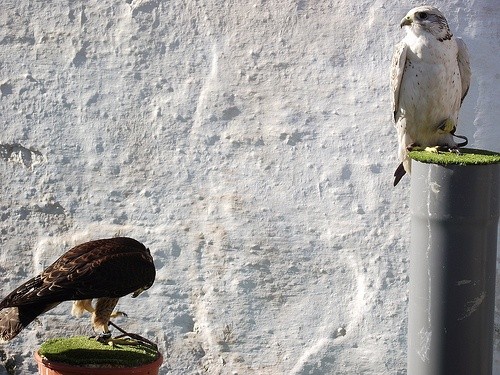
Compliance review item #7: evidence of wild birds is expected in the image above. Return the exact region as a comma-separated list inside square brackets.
[389, 4, 472, 187]
[0, 237, 158, 351]
